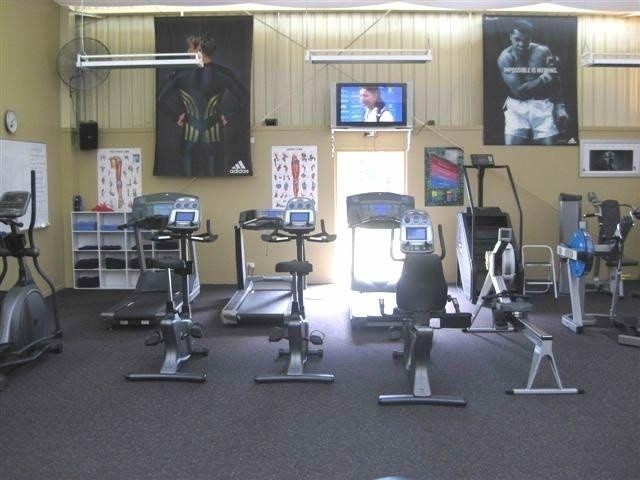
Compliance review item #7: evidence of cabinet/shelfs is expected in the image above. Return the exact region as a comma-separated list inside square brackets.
[71, 211, 194, 291]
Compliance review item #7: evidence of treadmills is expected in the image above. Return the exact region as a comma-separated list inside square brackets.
[99, 192, 200, 328]
[346, 192, 414, 327]
[221, 210, 295, 324]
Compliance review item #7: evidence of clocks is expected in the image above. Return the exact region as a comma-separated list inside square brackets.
[4, 111, 17, 134]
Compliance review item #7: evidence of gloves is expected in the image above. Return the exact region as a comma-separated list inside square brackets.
[554, 104, 568, 130]
[541, 56, 560, 85]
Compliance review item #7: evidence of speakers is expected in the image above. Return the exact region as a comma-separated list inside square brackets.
[79, 121, 99, 150]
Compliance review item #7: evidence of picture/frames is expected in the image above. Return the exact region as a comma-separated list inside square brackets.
[580, 140, 640, 177]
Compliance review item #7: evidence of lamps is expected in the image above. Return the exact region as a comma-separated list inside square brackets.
[75, 0, 204, 70]
[580, 0, 640, 67]
[304, 0, 431, 63]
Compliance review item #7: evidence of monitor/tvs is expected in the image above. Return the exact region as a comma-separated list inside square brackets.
[334, 82, 407, 129]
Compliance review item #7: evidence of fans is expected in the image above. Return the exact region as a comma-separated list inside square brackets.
[56, 36, 112, 129]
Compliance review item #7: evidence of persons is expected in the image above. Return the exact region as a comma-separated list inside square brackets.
[497, 20, 569, 145]
[157, 32, 249, 176]
[360, 86, 394, 122]
[590, 151, 616, 170]
